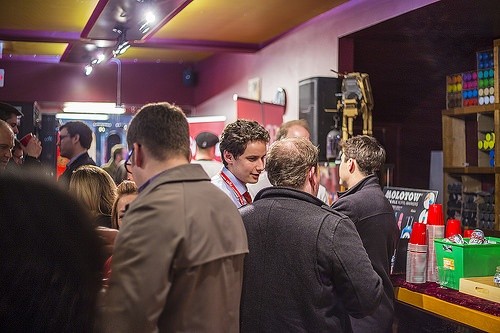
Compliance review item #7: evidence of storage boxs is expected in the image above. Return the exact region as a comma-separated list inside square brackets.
[432, 236, 500, 291]
[444, 48, 495, 231]
[459, 276, 500, 304]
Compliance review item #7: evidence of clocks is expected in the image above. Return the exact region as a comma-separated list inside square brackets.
[274, 88, 287, 114]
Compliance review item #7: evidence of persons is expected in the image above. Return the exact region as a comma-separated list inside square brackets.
[330, 136, 401, 333]
[0, 103, 383, 333]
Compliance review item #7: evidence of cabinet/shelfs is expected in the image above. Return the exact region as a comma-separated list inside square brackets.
[441, 39, 500, 238]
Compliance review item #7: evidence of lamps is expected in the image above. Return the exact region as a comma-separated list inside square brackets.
[83, 58, 99, 76]
[112, 25, 131, 56]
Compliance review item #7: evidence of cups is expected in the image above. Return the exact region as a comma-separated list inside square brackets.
[445, 219, 462, 239]
[426, 204, 444, 282]
[463, 229, 474, 238]
[406, 222, 428, 283]
[20, 132, 33, 147]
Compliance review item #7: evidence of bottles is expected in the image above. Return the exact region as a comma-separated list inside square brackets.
[477, 132, 495, 167]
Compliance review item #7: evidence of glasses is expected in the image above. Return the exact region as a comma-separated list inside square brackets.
[125, 144, 142, 174]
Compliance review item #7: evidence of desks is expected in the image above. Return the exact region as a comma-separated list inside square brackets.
[393, 275, 500, 333]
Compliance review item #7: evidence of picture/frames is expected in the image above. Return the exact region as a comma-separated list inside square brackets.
[248, 76, 261, 101]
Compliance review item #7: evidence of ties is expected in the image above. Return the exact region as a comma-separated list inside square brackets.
[243, 192, 252, 204]
